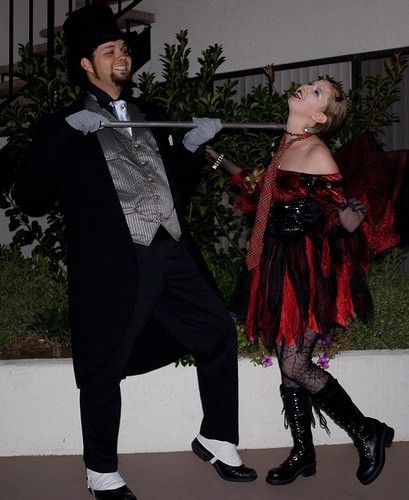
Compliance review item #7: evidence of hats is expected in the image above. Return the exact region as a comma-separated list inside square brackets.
[62, 2, 130, 58]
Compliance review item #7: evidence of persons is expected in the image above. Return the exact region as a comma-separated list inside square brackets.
[13, 3, 257, 500]
[202, 75, 409, 485]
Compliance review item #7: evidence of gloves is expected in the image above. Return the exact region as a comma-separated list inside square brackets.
[181, 117, 223, 153]
[65, 109, 111, 136]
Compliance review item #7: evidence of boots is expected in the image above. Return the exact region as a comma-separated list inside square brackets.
[311, 371, 394, 486]
[266, 385, 317, 485]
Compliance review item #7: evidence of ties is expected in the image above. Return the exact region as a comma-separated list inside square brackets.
[87, 91, 132, 136]
[246, 131, 315, 271]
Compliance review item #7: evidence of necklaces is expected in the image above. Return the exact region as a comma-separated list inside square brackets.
[281, 128, 311, 138]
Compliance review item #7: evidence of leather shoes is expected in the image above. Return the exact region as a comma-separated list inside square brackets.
[85, 477, 137, 500]
[192, 437, 257, 482]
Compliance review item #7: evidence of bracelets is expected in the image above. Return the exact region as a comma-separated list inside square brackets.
[212, 153, 224, 170]
